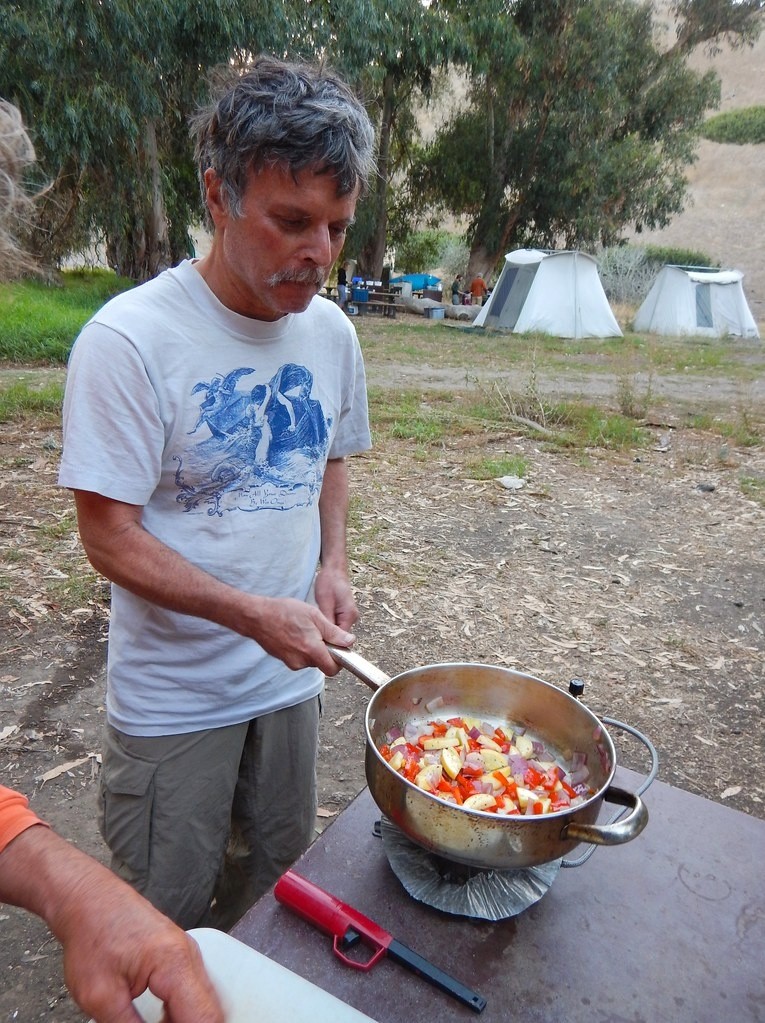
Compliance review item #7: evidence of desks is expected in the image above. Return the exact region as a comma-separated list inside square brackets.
[323, 285, 401, 315]
[228, 764, 765, 1023]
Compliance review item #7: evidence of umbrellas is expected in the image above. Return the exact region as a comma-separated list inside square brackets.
[388, 274, 441, 291]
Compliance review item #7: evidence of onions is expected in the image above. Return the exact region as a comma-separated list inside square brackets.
[385, 697, 610, 818]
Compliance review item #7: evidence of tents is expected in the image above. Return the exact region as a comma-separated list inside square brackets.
[472, 249, 624, 339]
[632, 264, 760, 339]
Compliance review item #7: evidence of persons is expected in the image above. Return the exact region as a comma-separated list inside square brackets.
[464, 272, 487, 306]
[337, 262, 353, 310]
[450, 274, 463, 305]
[0, 785, 227, 1023]
[57, 55, 375, 934]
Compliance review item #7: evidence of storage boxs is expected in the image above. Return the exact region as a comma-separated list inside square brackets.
[424, 308, 445, 319]
[350, 288, 369, 302]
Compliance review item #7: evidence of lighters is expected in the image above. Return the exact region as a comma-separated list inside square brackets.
[273, 870, 487, 1014]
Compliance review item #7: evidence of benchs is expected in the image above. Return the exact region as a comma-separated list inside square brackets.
[319, 293, 339, 303]
[352, 301, 405, 319]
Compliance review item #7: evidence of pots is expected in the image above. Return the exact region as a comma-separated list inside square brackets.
[323, 637, 648, 872]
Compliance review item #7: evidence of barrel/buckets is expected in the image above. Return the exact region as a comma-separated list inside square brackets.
[352, 289, 368, 302]
[423, 307, 445, 319]
[347, 306, 358, 316]
